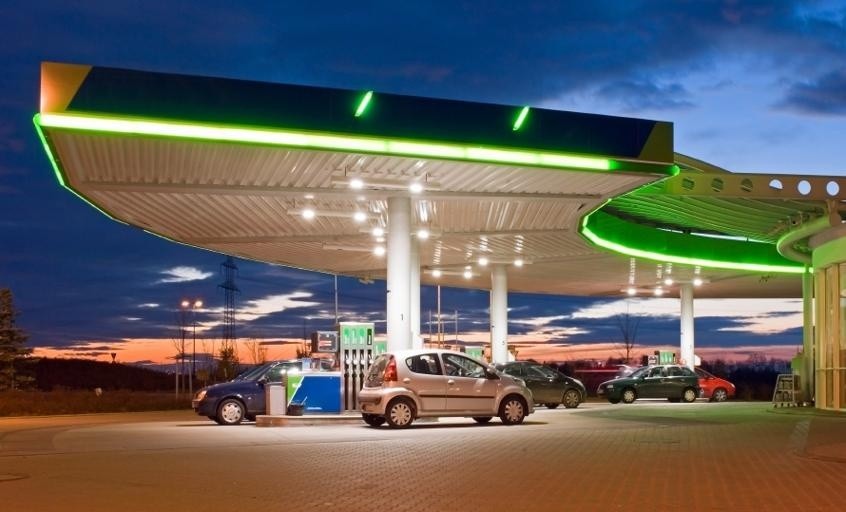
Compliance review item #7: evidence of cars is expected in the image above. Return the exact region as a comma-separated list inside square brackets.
[473, 361, 587, 408]
[192, 359, 333, 425]
[616, 362, 736, 402]
[596, 364, 699, 404]
[358, 348, 535, 429]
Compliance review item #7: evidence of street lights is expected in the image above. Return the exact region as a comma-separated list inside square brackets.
[182, 299, 203, 398]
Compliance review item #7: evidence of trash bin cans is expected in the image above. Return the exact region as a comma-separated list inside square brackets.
[264, 381, 288, 416]
